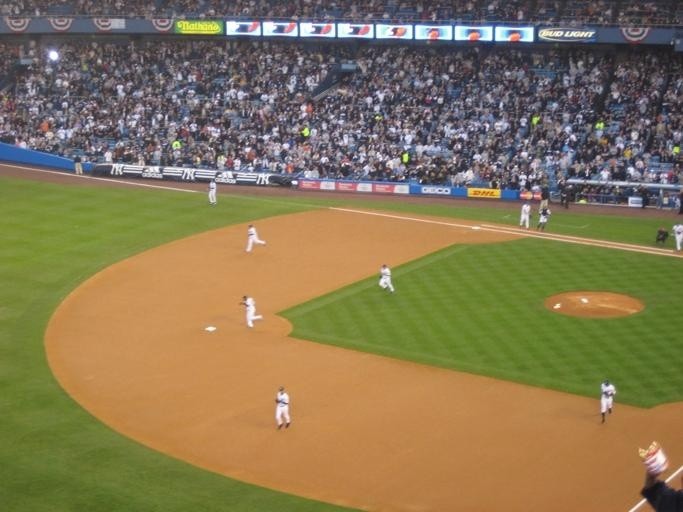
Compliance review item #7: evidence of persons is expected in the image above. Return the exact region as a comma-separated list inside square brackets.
[672, 223, 682, 252]
[1, 1, 683, 208]
[641, 465, 683, 512]
[239, 296, 264, 328]
[378, 264, 395, 293]
[538, 187, 550, 214]
[244, 225, 268, 253]
[536, 205, 551, 230]
[275, 386, 291, 428]
[600, 380, 617, 423]
[656, 228, 668, 242]
[519, 201, 531, 230]
